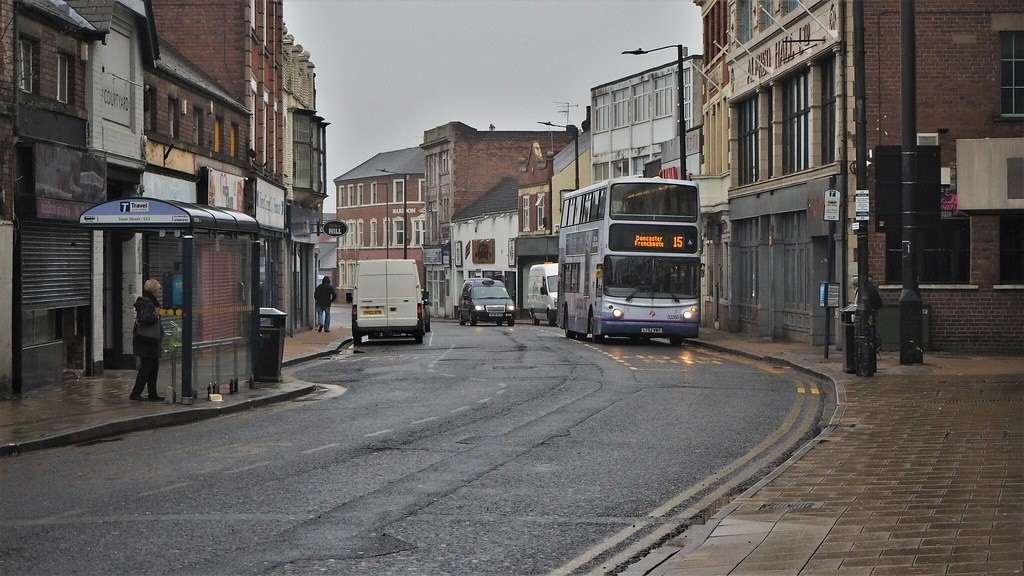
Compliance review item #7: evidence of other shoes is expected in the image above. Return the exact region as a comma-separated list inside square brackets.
[324, 330, 330, 332]
[318, 325, 323, 332]
[129, 393, 148, 401]
[148, 393, 165, 401]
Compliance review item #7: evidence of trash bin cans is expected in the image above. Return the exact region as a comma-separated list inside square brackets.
[454, 305, 459, 320]
[250, 306, 288, 383]
[839, 304, 859, 374]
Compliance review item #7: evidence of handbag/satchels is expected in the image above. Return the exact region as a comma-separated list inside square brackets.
[136, 316, 160, 344]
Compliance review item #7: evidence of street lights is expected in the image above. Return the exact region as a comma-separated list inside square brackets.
[620, 44, 689, 181]
[377, 167, 409, 259]
[537, 120, 580, 190]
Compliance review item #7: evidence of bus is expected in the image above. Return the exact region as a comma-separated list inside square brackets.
[557, 176, 715, 347]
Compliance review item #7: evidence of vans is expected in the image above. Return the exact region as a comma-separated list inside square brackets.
[458, 278, 516, 327]
[345, 259, 429, 344]
[526, 262, 560, 326]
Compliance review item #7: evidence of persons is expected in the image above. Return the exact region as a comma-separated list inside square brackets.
[850, 277, 881, 361]
[128, 279, 165, 401]
[314, 276, 337, 332]
